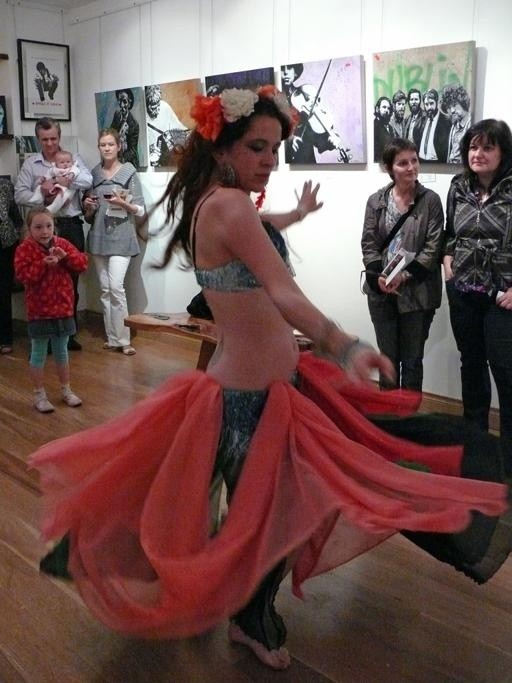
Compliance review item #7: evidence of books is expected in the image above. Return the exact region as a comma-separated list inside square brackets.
[105, 189, 134, 219]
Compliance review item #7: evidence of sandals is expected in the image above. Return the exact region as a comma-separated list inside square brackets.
[122, 346, 136, 355]
[103, 341, 114, 349]
[0, 345, 13, 355]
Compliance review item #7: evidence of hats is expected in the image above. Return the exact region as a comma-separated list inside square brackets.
[115, 89, 134, 109]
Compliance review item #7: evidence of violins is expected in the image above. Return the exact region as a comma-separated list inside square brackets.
[290, 84, 353, 164]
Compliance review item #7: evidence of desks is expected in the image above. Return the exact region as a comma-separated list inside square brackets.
[123, 311, 317, 371]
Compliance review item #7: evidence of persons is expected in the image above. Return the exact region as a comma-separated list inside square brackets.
[443, 118, 512, 480]
[33, 61, 59, 102]
[80, 128, 147, 356]
[14, 205, 89, 414]
[373, 97, 399, 159]
[26, 152, 79, 215]
[280, 64, 341, 163]
[28, 82, 512, 670]
[0, 100, 6, 134]
[415, 89, 450, 162]
[389, 91, 407, 140]
[403, 87, 423, 142]
[145, 85, 191, 166]
[361, 137, 445, 391]
[14, 117, 93, 362]
[441, 81, 472, 165]
[0, 175, 22, 353]
[111, 88, 140, 168]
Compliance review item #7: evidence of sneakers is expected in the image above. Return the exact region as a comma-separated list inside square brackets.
[67, 338, 81, 350]
[33, 395, 55, 412]
[62, 391, 82, 407]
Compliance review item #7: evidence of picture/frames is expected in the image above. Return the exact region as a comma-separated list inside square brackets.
[93, 85, 149, 171]
[204, 67, 279, 169]
[16, 38, 72, 121]
[0, 95, 10, 142]
[280, 54, 368, 167]
[372, 40, 476, 162]
[144, 78, 205, 168]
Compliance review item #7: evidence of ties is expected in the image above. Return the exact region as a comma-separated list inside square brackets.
[421, 116, 433, 157]
